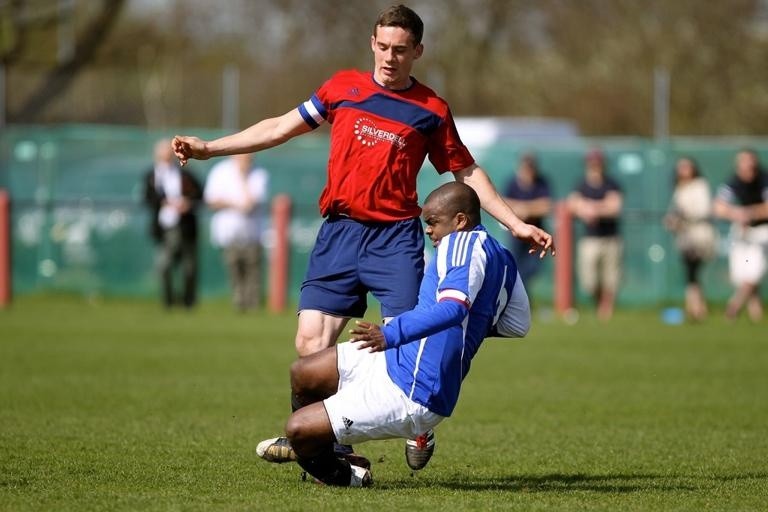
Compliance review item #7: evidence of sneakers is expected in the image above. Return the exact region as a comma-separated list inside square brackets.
[315, 449, 371, 488]
[405, 428, 436, 471]
[255, 436, 296, 464]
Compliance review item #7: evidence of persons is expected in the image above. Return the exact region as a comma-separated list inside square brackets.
[713, 148, 768, 327]
[503, 157, 556, 287]
[140, 138, 206, 310]
[195, 154, 272, 314]
[247, 180, 535, 487]
[169, 1, 556, 472]
[563, 148, 625, 320]
[661, 155, 721, 322]
